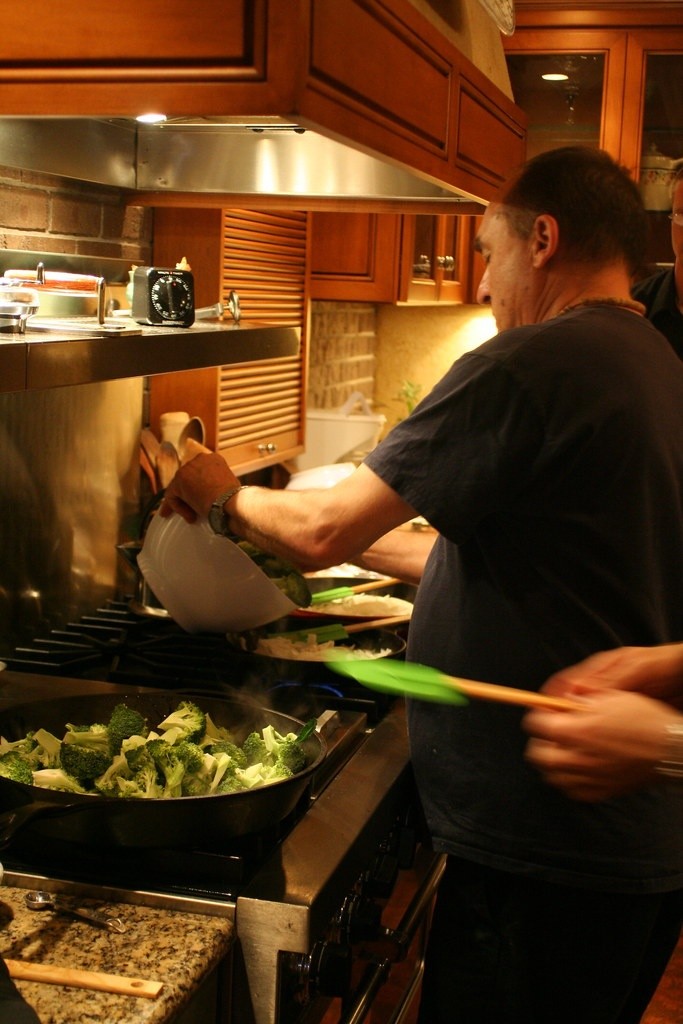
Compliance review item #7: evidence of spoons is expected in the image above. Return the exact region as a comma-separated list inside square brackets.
[22, 889, 125, 933]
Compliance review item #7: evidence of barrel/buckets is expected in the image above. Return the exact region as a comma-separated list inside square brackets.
[297, 391, 386, 472]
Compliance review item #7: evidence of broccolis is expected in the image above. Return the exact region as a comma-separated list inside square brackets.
[0, 699, 318, 797]
[233, 540, 313, 608]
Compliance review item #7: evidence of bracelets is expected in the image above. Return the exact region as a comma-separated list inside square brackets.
[655, 719, 682, 780]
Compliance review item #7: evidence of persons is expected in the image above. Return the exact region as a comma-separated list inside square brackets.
[522, 642, 683, 806]
[159, 144, 683, 1024]
[630, 159, 682, 360]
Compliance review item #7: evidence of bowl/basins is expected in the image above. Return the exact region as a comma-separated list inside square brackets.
[136, 500, 298, 644]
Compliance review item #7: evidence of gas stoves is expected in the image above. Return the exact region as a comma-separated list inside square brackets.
[6, 594, 413, 984]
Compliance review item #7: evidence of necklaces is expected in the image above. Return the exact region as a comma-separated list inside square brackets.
[553, 296, 646, 316]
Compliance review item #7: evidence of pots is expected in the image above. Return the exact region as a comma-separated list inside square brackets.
[0, 688, 337, 848]
[287, 576, 420, 620]
[234, 622, 409, 689]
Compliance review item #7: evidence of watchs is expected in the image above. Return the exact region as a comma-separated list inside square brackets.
[207, 483, 248, 537]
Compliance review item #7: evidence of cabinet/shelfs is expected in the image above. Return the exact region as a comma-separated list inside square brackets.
[1, 1, 683, 476]
